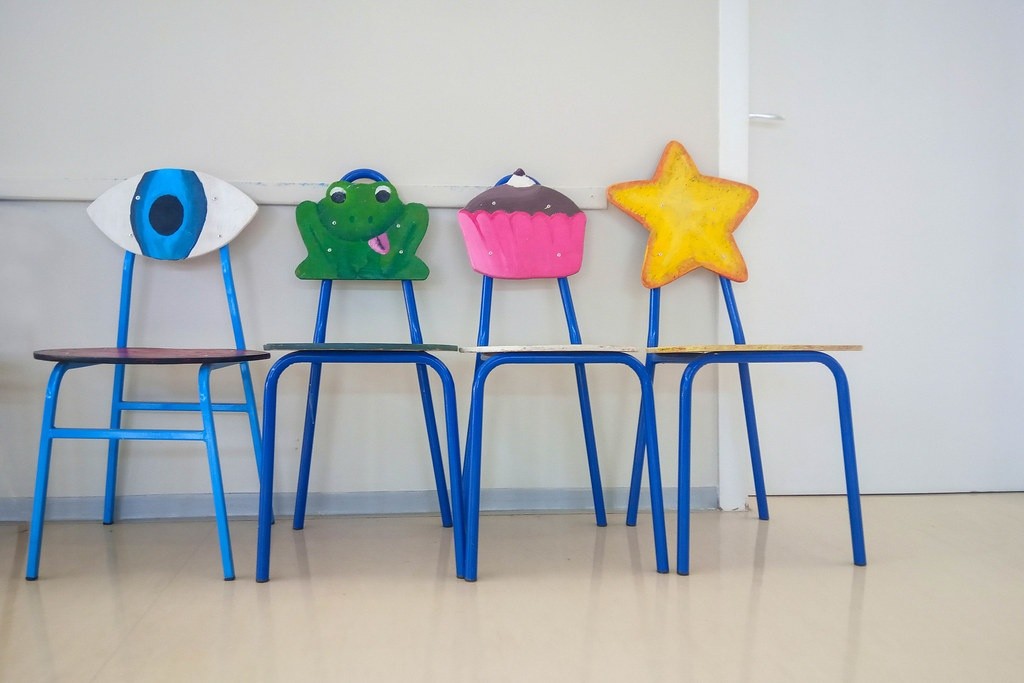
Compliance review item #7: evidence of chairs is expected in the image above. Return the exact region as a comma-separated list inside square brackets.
[26, 167, 274, 582]
[458, 169, 668, 581]
[254, 167, 464, 587]
[604, 142, 866, 577]
